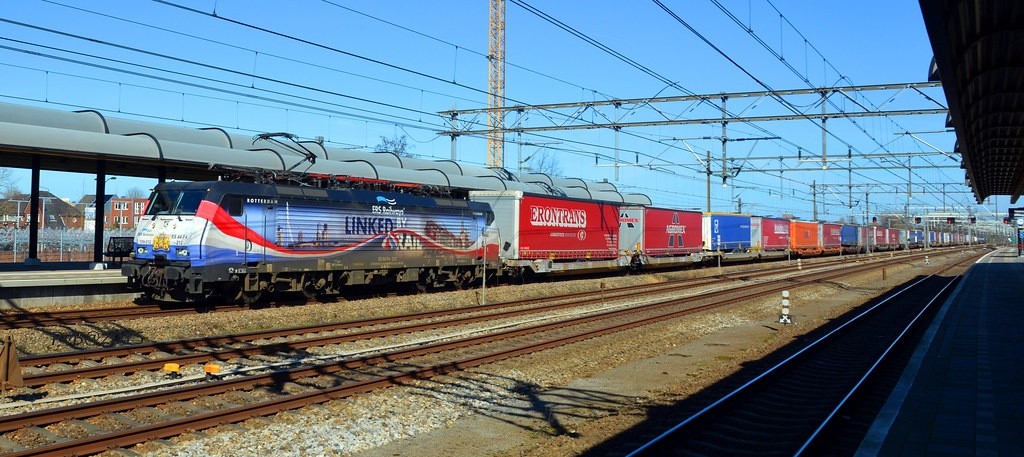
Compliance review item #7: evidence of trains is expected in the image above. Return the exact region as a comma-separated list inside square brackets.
[121, 181, 986, 313]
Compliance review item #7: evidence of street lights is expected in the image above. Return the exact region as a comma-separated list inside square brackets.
[72, 201, 86, 253]
[56, 226, 65, 262]
[13, 222, 18, 263]
[39, 197, 57, 230]
[8, 200, 30, 229]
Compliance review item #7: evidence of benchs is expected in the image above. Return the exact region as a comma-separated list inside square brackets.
[103, 236, 134, 262]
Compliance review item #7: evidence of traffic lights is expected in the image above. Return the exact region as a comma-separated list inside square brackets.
[968, 218, 976, 223]
[947, 218, 955, 224]
[915, 217, 921, 224]
[1003, 217, 1009, 224]
[873, 217, 877, 223]
